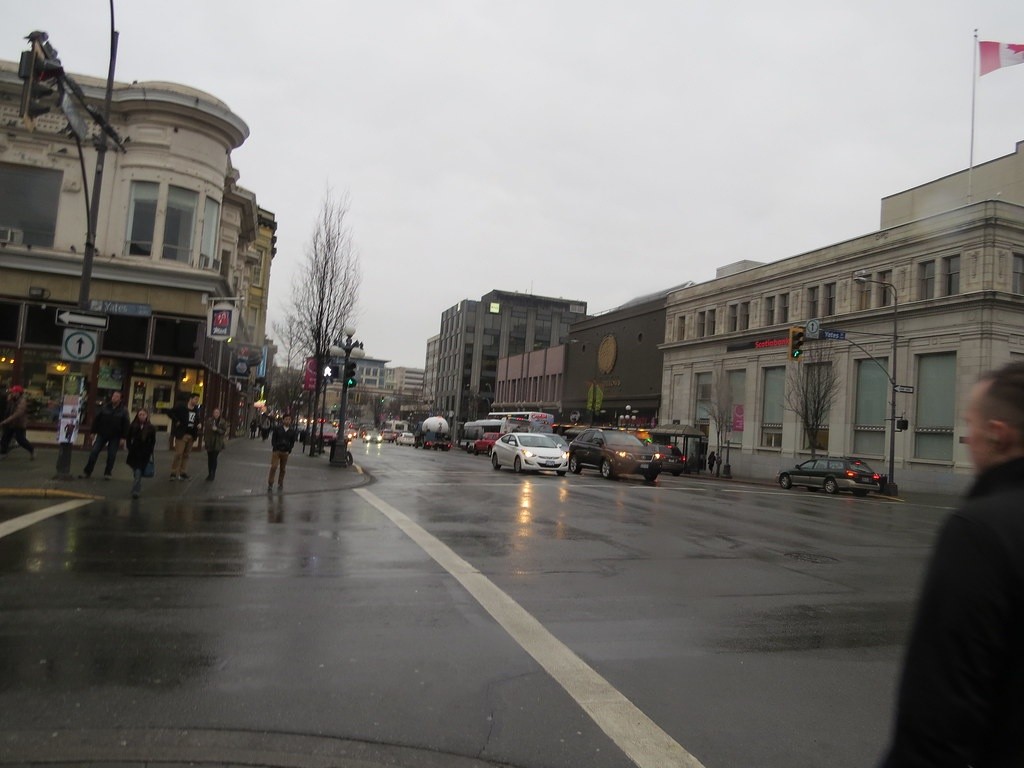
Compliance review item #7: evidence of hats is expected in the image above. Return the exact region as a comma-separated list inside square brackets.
[8, 385, 24, 393]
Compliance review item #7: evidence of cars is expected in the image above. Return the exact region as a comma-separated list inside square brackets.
[491, 433, 569, 475]
[396, 433, 415, 446]
[660, 445, 685, 476]
[779, 457, 881, 495]
[303, 418, 382, 446]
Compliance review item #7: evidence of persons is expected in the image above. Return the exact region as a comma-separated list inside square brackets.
[204, 407, 228, 481]
[268, 414, 296, 490]
[78, 390, 129, 480]
[167, 393, 202, 481]
[880, 356, 1024, 768]
[0, 384, 38, 460]
[64, 421, 75, 442]
[250, 412, 280, 440]
[686, 451, 717, 473]
[127, 408, 156, 499]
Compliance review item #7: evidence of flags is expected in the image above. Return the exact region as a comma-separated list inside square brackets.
[979, 41, 1024, 76]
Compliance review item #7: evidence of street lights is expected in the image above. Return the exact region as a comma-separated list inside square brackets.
[859, 277, 897, 496]
[330, 326, 366, 464]
[620, 405, 636, 431]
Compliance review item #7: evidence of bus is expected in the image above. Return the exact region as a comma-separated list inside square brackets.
[487, 411, 555, 434]
[461, 418, 508, 446]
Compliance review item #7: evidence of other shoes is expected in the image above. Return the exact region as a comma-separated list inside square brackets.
[132, 490, 142, 502]
[169, 474, 192, 482]
[78, 473, 91, 479]
[104, 474, 110, 480]
[205, 474, 215, 481]
[0, 451, 7, 460]
[268, 484, 283, 490]
[31, 448, 38, 461]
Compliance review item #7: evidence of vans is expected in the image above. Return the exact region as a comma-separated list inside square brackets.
[381, 420, 408, 443]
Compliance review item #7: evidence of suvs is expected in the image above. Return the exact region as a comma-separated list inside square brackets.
[569, 428, 662, 482]
[474, 432, 504, 457]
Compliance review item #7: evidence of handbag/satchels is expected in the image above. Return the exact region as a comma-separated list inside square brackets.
[141, 452, 155, 477]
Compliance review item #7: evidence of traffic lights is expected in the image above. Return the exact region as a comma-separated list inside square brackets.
[897, 420, 908, 430]
[788, 325, 806, 362]
[382, 396, 384, 402]
[324, 362, 357, 388]
[17, 39, 64, 133]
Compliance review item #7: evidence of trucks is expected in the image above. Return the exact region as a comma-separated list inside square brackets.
[414, 416, 451, 451]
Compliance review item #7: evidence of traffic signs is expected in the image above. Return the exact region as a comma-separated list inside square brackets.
[894, 385, 914, 394]
